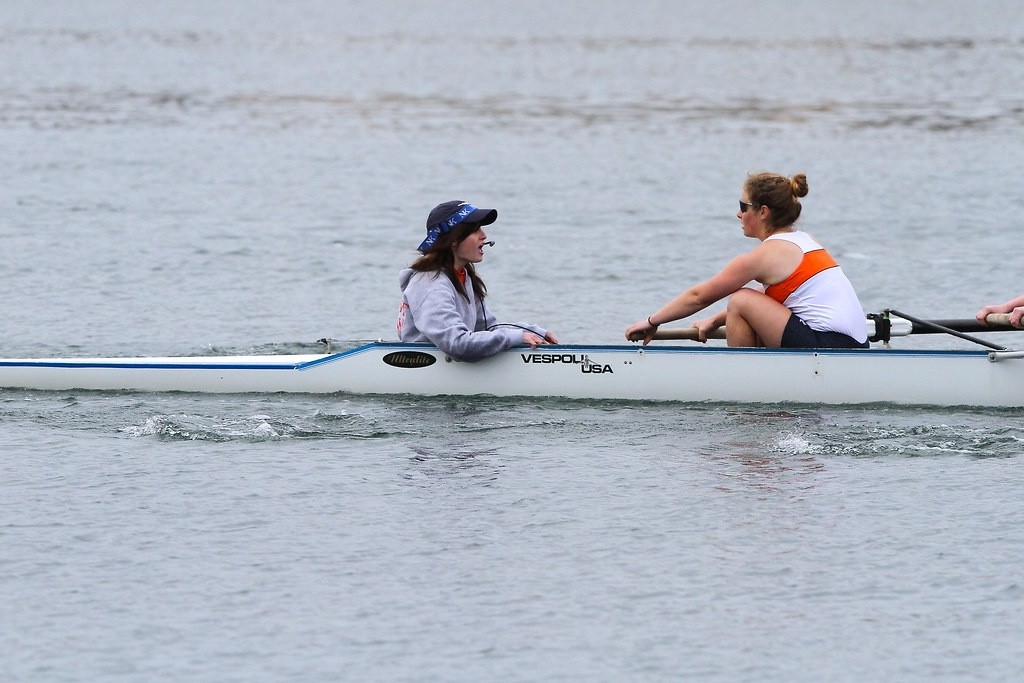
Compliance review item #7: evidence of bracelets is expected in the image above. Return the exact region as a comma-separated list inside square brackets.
[648, 316, 660, 327]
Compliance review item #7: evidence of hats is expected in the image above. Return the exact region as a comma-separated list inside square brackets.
[427, 199, 498, 237]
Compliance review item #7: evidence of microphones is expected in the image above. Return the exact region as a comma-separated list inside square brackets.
[484, 240, 495, 248]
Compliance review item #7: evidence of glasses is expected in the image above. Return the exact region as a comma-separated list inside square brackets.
[740, 201, 758, 213]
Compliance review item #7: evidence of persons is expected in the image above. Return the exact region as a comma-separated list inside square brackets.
[625, 172, 870, 350]
[975, 293, 1024, 330]
[396, 200, 558, 363]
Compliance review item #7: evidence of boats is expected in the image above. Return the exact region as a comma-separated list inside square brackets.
[0, 329, 1024, 414]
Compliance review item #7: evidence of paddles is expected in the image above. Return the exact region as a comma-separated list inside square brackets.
[630, 311, 1024, 349]
